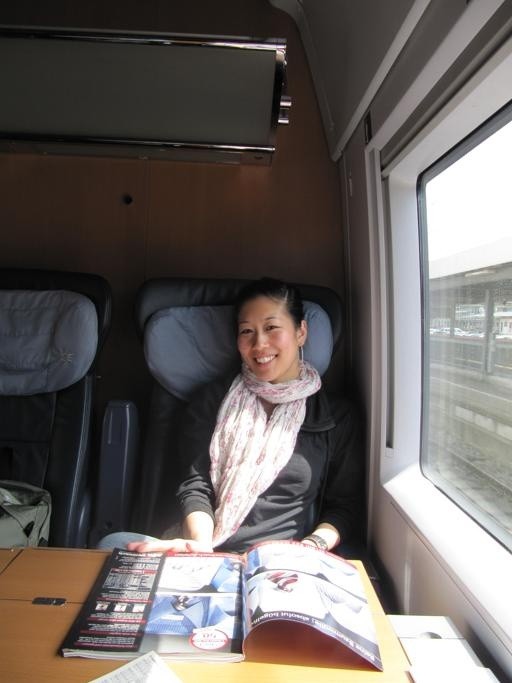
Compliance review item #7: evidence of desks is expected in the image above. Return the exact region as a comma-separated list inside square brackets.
[0, 542, 422, 683]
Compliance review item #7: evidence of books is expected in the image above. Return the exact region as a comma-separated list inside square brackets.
[58, 544, 386, 673]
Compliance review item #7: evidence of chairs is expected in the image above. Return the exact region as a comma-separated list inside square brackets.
[2, 268, 112, 551]
[92, 271, 353, 550]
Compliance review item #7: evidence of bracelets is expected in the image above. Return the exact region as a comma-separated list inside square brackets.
[302, 531, 329, 551]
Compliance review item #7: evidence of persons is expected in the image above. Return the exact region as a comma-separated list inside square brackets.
[123, 274, 367, 553]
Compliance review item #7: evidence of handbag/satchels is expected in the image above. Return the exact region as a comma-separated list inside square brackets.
[0, 479, 52, 548]
